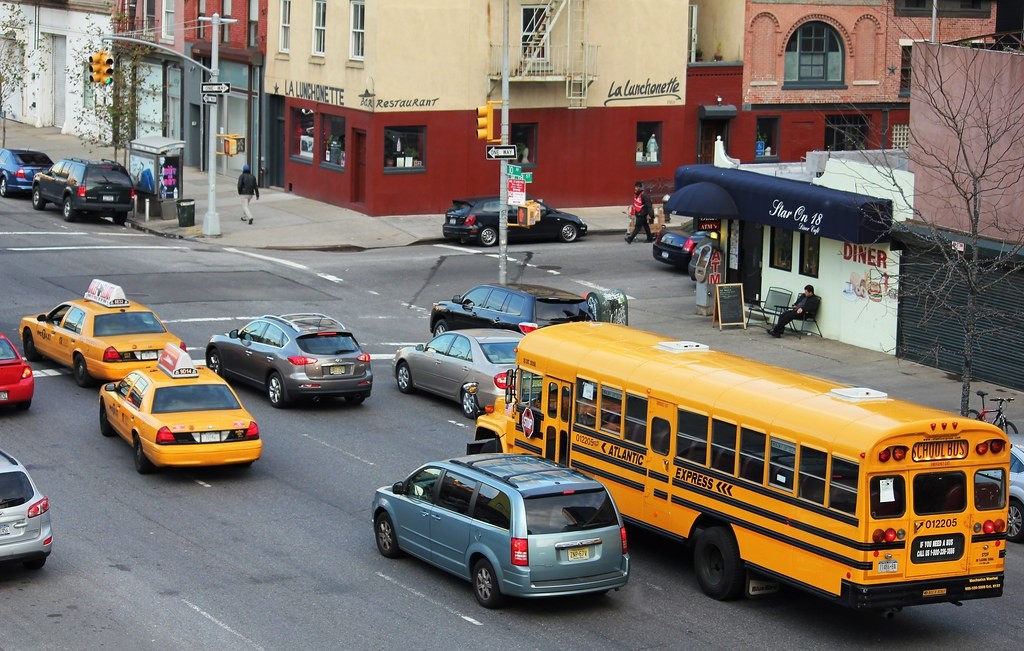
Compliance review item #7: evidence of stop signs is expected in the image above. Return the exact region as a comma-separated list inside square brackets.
[521, 408, 535, 439]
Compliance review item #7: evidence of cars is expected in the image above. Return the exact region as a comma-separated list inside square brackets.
[0, 147, 55, 198]
[0, 446, 54, 571]
[442, 193, 589, 247]
[0, 332, 34, 412]
[945, 433, 1024, 544]
[390, 326, 554, 422]
[17, 278, 188, 392]
[654, 220, 715, 281]
[205, 311, 374, 411]
[429, 282, 596, 339]
[97, 341, 263, 479]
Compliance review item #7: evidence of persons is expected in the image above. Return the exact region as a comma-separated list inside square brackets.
[131, 161, 154, 192]
[625, 182, 654, 243]
[767, 285, 817, 338]
[237, 165, 259, 225]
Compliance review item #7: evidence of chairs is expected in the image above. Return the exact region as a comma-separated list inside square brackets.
[632, 418, 1001, 517]
[747, 287, 793, 330]
[779, 293, 823, 339]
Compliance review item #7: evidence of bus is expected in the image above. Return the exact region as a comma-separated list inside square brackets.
[458, 319, 1014, 624]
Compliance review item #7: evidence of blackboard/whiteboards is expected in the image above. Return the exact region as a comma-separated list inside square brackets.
[712, 283, 746, 325]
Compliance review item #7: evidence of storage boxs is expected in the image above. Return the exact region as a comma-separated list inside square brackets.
[630, 205, 665, 234]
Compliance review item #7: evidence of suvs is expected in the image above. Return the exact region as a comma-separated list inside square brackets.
[31, 157, 136, 225]
[369, 451, 632, 611]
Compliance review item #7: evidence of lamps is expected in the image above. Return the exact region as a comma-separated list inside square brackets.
[359, 76, 375, 113]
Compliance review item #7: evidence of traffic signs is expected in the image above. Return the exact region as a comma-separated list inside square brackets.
[201, 94, 218, 105]
[200, 81, 231, 95]
[485, 144, 518, 160]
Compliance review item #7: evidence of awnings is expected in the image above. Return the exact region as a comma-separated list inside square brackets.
[675, 164, 893, 244]
[664, 182, 738, 218]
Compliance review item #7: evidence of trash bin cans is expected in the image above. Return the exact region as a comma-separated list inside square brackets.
[176, 199, 195, 227]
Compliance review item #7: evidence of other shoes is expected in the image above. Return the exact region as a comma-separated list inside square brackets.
[644, 239, 653, 243]
[625, 237, 631, 244]
[249, 218, 253, 224]
[767, 329, 781, 337]
[241, 216, 246, 221]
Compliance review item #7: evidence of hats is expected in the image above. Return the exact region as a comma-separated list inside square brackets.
[243, 165, 250, 170]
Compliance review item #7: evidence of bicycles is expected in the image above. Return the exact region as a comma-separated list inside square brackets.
[967, 390, 1019, 435]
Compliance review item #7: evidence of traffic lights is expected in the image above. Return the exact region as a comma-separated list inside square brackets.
[223, 138, 238, 156]
[475, 105, 494, 141]
[100, 51, 114, 86]
[89, 50, 104, 82]
[232, 135, 246, 153]
